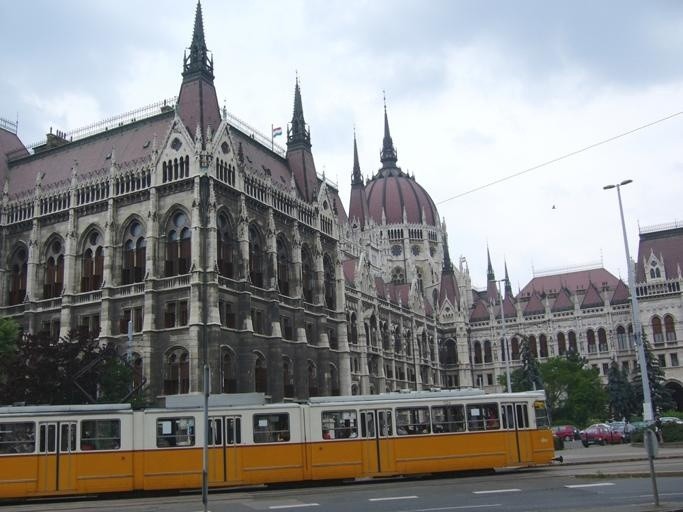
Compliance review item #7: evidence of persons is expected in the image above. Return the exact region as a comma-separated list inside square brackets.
[276, 426, 288, 441]
[322, 426, 358, 439]
[396, 408, 497, 435]
[81, 436, 119, 450]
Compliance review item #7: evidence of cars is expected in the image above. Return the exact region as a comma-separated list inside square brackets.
[551, 416, 683, 450]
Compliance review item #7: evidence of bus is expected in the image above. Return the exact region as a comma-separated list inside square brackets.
[0, 387, 556, 502]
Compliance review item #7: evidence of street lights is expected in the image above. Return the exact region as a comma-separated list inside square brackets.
[602, 178, 672, 428]
